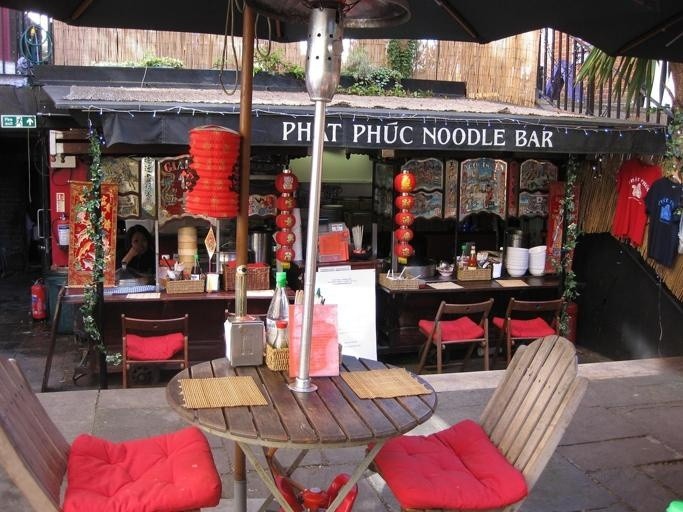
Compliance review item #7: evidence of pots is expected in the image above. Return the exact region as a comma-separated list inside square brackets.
[246, 226, 275, 266]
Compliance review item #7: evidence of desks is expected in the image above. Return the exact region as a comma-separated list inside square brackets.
[38, 282, 299, 392]
[376, 272, 564, 356]
[163, 349, 439, 512]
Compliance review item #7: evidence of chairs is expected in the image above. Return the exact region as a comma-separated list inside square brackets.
[0, 351, 222, 512]
[119, 312, 190, 388]
[414, 296, 495, 375]
[361, 332, 589, 511]
[490, 296, 564, 370]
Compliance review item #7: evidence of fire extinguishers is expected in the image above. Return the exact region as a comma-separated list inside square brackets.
[51, 214, 70, 252]
[30, 277, 47, 320]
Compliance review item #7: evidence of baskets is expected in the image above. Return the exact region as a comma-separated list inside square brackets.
[162, 276, 205, 295]
[222, 260, 271, 292]
[266, 341, 343, 372]
[377, 271, 420, 292]
[456, 259, 493, 281]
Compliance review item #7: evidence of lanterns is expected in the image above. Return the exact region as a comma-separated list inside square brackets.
[393, 171, 417, 264]
[184, 126, 242, 219]
[275, 169, 298, 269]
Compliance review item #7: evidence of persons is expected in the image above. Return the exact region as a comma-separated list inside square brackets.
[116, 225, 155, 277]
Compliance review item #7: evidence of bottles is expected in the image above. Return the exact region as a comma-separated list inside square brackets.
[267, 271, 287, 343]
[456, 245, 476, 270]
[274, 320, 287, 348]
[183, 272, 188, 280]
[190, 255, 201, 280]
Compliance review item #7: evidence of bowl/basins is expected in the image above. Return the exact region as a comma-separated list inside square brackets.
[506, 245, 547, 277]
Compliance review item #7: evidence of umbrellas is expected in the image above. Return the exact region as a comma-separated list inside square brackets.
[1, 0, 682, 511]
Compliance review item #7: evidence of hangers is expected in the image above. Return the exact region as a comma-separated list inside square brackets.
[665, 164, 682, 185]
[635, 153, 656, 166]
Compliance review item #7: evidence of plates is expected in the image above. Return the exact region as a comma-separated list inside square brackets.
[320, 204, 345, 209]
[439, 270, 453, 277]
